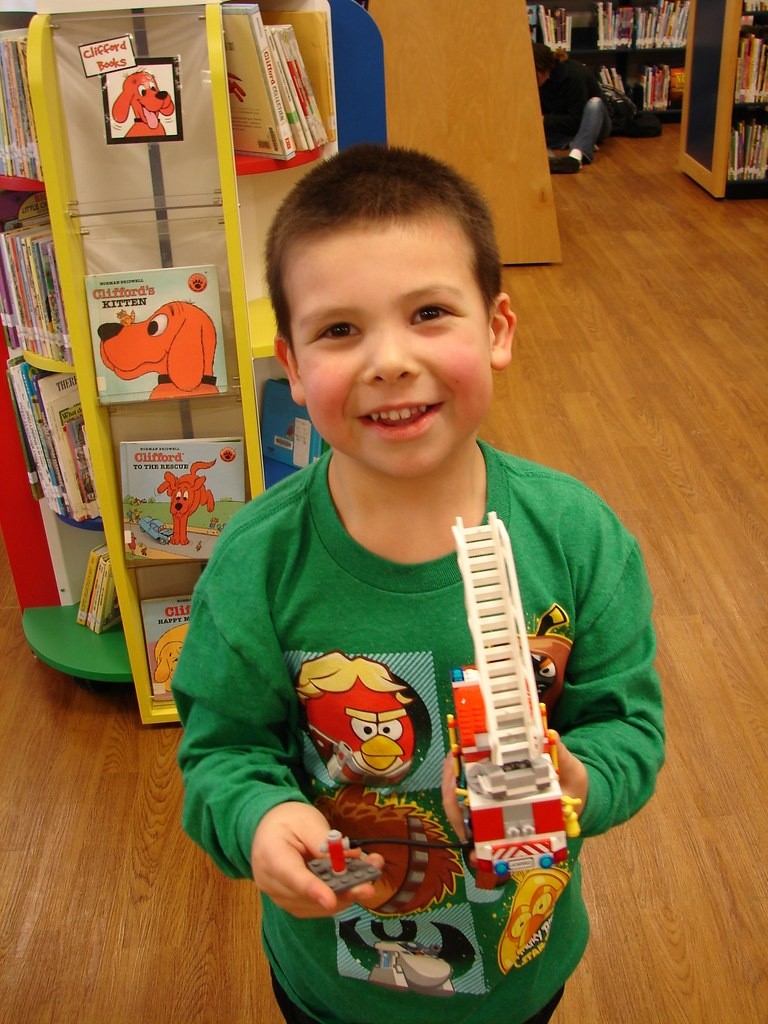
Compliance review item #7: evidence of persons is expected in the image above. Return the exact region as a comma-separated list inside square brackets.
[171, 144, 665, 1023]
[531, 43, 613, 175]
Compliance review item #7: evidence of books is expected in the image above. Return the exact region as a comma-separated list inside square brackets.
[140, 595, 192, 701]
[1, 38, 121, 635]
[527, 1, 768, 181]
[222, 3, 337, 160]
[260, 376, 331, 469]
[120, 437, 245, 561]
[85, 264, 229, 404]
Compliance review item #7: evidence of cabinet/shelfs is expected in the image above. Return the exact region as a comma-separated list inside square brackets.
[0, 8, 343, 724]
[536, 2, 768, 201]
